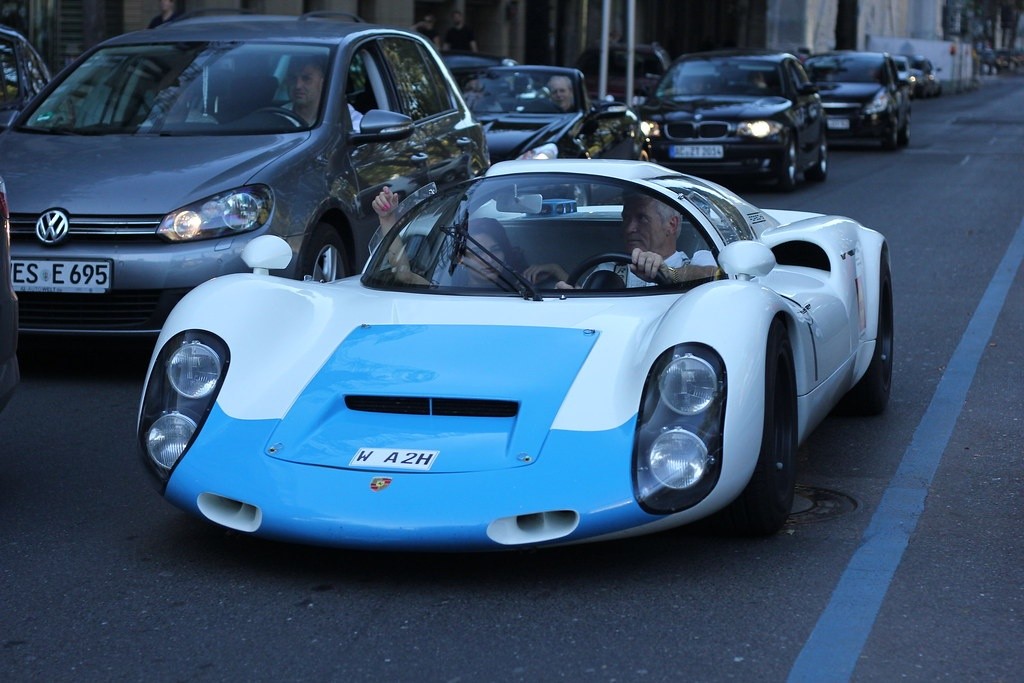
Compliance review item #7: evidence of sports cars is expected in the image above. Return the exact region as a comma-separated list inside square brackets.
[134, 158, 895, 555]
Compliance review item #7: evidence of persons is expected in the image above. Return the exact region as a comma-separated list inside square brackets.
[271, 52, 364, 134]
[546, 76, 574, 114]
[553, 184, 726, 291]
[371, 186, 579, 292]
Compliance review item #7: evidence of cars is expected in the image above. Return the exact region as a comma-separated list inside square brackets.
[567, 40, 671, 105]
[630, 48, 829, 193]
[891, 55, 943, 99]
[453, 62, 653, 182]
[0, 11, 492, 343]
[436, 49, 504, 83]
[797, 50, 913, 150]
[0, 25, 53, 131]
